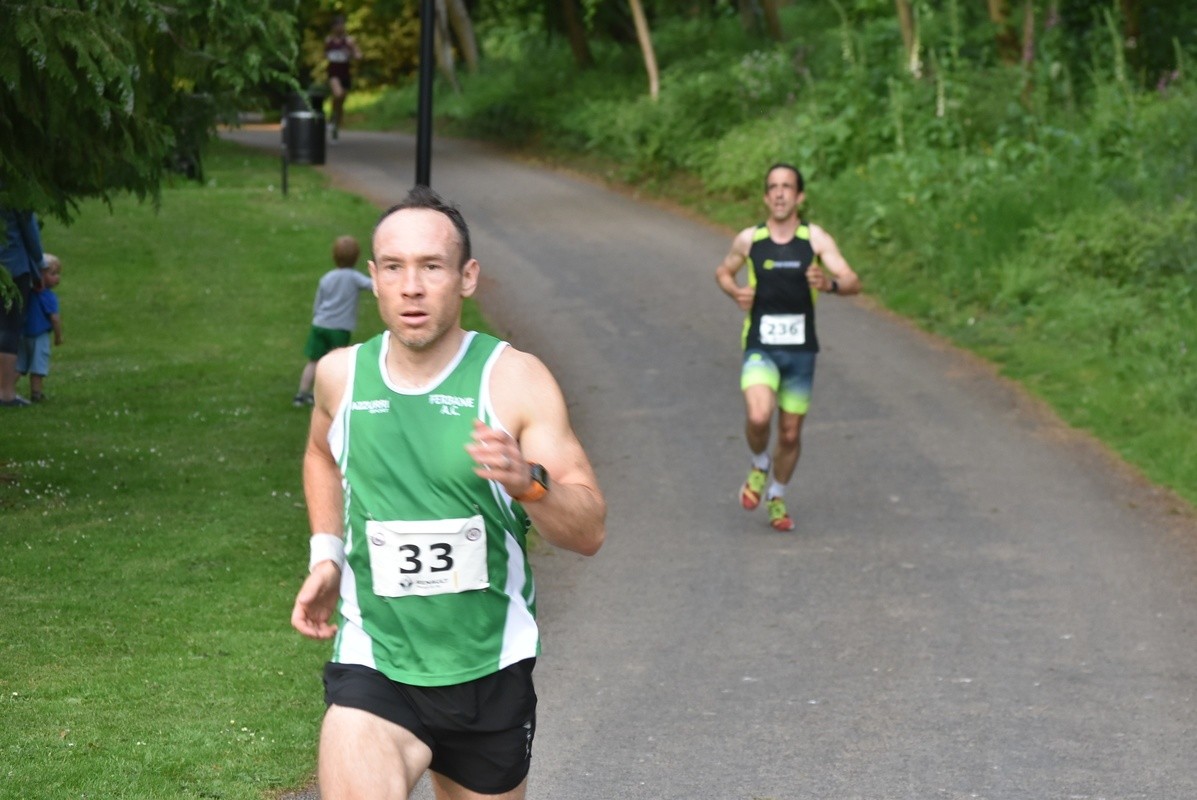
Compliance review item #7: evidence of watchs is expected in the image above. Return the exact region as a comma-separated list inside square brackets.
[512, 461, 549, 503]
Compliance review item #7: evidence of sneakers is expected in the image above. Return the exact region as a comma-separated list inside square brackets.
[739, 455, 771, 509]
[766, 497, 795, 533]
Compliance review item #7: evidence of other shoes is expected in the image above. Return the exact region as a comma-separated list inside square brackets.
[326, 122, 335, 130]
[330, 137, 338, 146]
[30, 393, 45, 403]
[294, 394, 314, 408]
[0, 392, 28, 407]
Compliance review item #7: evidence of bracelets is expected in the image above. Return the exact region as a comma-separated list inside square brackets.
[305, 535, 346, 572]
[828, 281, 838, 295]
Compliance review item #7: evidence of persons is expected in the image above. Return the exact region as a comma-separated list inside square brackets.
[716, 163, 864, 531]
[292, 235, 373, 404]
[0, 211, 63, 407]
[324, 9, 362, 145]
[291, 199, 606, 800]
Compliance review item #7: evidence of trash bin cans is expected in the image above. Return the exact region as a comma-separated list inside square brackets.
[287, 90, 327, 165]
